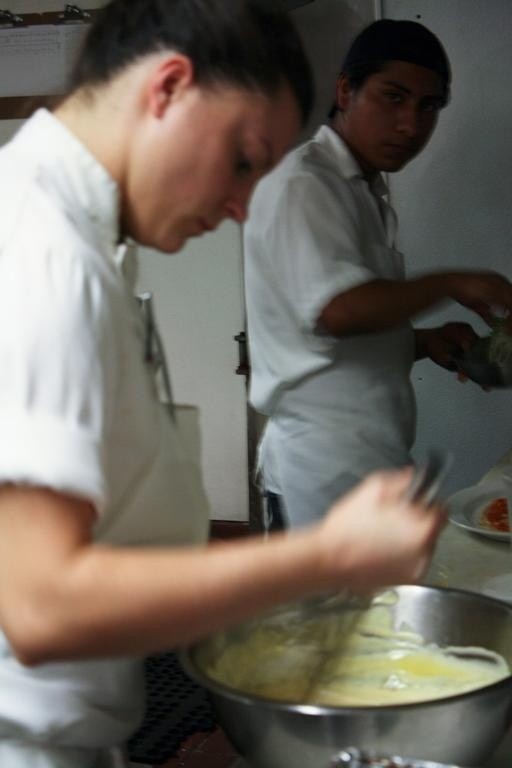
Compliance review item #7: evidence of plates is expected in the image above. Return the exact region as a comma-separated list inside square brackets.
[443, 480, 512, 544]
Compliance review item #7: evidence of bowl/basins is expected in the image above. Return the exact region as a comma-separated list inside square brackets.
[177, 579, 511, 767]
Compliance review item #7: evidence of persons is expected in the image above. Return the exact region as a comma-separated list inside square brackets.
[0, 0, 451, 767]
[242, 17, 512, 543]
